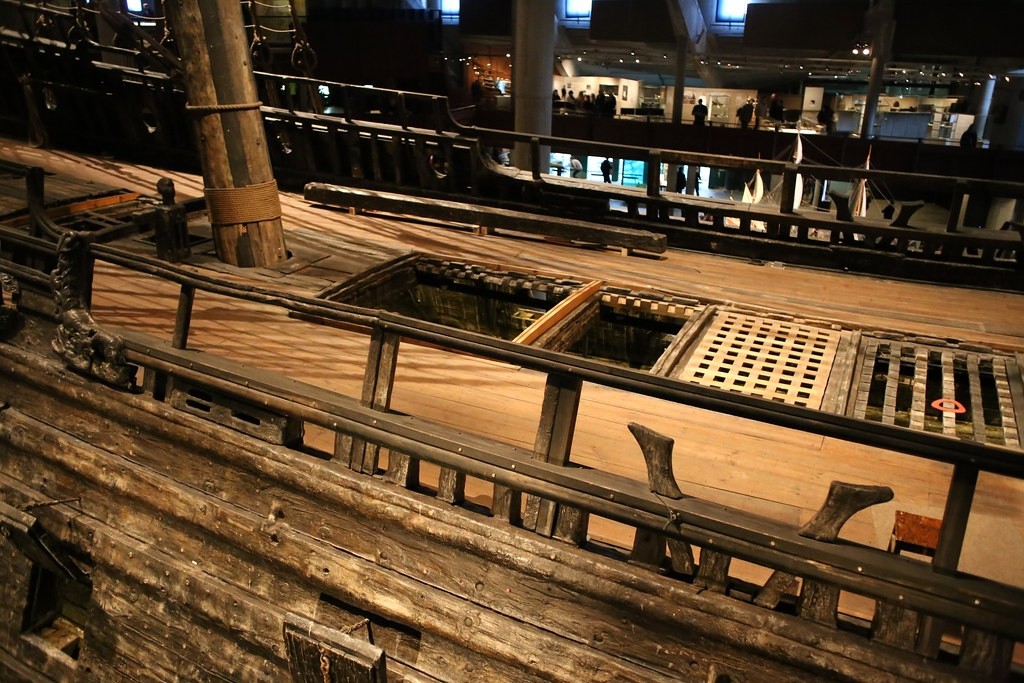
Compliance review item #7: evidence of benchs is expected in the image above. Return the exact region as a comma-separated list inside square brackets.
[305, 181, 668, 258]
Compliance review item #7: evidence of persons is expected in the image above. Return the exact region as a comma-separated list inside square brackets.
[691, 99, 708, 126]
[569, 156, 583, 178]
[600, 157, 612, 183]
[694, 167, 701, 196]
[866, 190, 872, 210]
[960, 123, 977, 147]
[881, 200, 895, 220]
[675, 166, 686, 193]
[471, 80, 480, 103]
[735, 98, 760, 129]
[552, 88, 616, 118]
